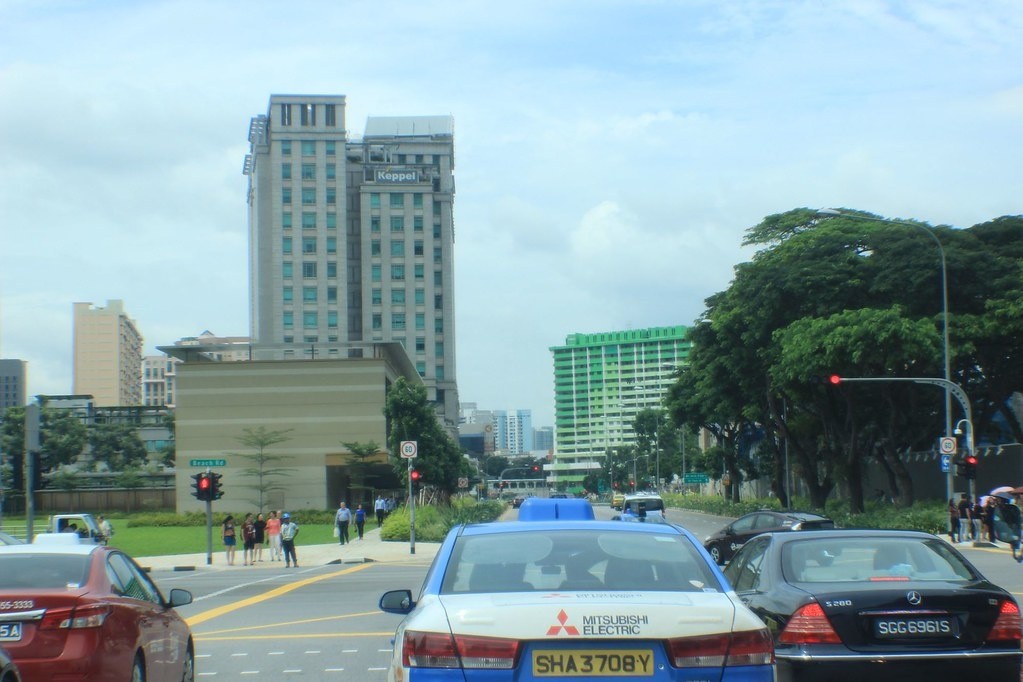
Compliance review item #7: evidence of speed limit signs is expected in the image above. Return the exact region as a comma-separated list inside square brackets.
[400, 441, 417, 458]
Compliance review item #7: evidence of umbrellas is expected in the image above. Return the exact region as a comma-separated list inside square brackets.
[990, 487, 1023, 500]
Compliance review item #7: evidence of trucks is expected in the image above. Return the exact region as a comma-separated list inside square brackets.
[18, 515, 106, 546]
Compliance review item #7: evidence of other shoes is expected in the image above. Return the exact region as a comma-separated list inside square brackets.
[340, 542, 344, 545]
[347, 540, 349, 543]
[359, 537, 363, 540]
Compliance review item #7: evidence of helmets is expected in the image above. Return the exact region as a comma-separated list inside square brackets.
[283, 513, 290, 519]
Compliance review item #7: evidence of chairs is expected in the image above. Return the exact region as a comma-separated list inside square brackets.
[603, 556, 655, 590]
[873, 547, 906, 570]
[470, 562, 527, 591]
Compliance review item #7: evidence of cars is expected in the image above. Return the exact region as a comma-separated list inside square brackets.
[722, 530, 1023, 682]
[381, 520, 779, 682]
[0, 532, 197, 682]
[703, 511, 835, 566]
[611, 495, 625, 508]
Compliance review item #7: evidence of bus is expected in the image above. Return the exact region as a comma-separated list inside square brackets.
[486, 480, 549, 502]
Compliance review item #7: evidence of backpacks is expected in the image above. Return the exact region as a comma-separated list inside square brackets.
[109, 524, 115, 535]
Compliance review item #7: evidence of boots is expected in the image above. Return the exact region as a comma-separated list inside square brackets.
[294, 561, 299, 567]
[286, 562, 290, 568]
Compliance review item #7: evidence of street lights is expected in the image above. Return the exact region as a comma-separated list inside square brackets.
[577, 416, 614, 493]
[618, 403, 637, 492]
[818, 208, 955, 528]
[634, 363, 686, 494]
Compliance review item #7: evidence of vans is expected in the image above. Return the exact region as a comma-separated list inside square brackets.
[620, 493, 666, 524]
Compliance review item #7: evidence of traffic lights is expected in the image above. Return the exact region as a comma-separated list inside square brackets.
[191, 472, 225, 501]
[411, 469, 419, 480]
[630, 481, 634, 486]
[967, 456, 977, 479]
[534, 465, 538, 471]
[9, 450, 51, 490]
[827, 368, 840, 393]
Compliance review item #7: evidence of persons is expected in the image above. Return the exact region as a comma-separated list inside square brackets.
[375, 494, 398, 527]
[334, 502, 353, 545]
[354, 504, 367, 540]
[221, 511, 299, 567]
[61, 523, 77, 533]
[97, 516, 111, 545]
[949, 495, 1023, 546]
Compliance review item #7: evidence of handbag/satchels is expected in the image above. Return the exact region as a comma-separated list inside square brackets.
[334, 526, 339, 537]
[354, 525, 357, 532]
